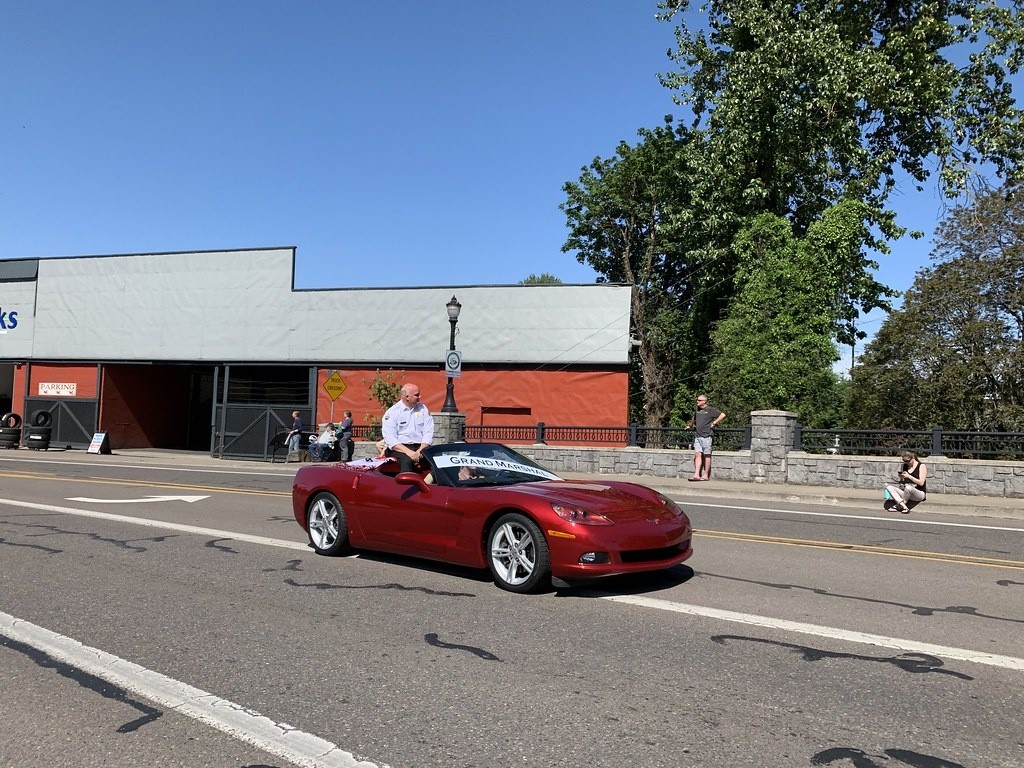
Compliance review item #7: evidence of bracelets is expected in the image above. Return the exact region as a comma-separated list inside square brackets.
[712, 422, 715, 425]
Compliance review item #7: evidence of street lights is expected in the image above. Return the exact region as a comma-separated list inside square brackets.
[440, 294, 462, 412]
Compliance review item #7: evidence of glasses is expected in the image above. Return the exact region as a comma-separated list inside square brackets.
[697, 400, 706, 401]
[903, 458, 913, 462]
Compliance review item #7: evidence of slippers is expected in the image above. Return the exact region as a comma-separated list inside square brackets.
[902, 509, 910, 514]
[889, 506, 898, 511]
[689, 476, 709, 481]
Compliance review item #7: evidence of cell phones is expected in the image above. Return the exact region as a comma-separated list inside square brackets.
[898, 472, 902, 474]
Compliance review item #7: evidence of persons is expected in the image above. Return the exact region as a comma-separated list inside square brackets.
[375, 439, 387, 457]
[685, 394, 726, 481]
[309, 423, 337, 462]
[458, 451, 485, 480]
[339, 410, 353, 463]
[382, 383, 435, 473]
[285, 411, 302, 463]
[887, 450, 927, 513]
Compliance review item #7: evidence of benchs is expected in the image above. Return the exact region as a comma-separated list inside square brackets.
[270, 430, 340, 462]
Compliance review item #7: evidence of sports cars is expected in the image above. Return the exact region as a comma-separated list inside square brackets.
[292, 442, 693, 594]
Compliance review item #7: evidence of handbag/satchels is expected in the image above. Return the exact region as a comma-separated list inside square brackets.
[334, 424, 344, 438]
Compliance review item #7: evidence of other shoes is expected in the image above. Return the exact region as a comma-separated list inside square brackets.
[314, 458, 322, 462]
[340, 460, 347, 463]
